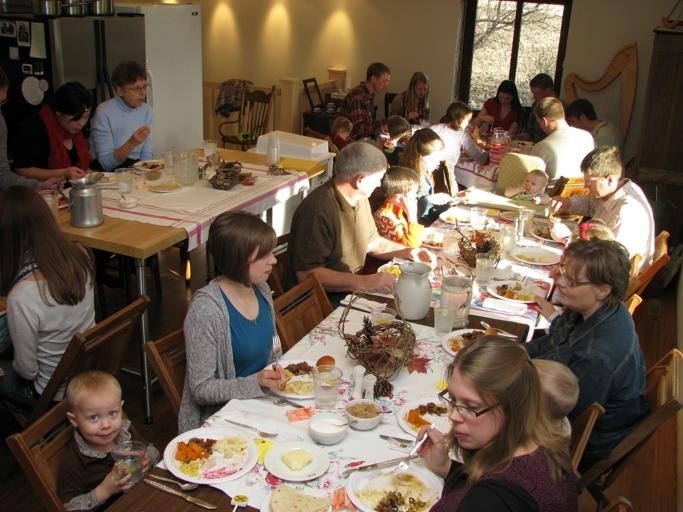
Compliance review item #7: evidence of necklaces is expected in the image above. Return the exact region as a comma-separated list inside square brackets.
[219, 278, 261, 325]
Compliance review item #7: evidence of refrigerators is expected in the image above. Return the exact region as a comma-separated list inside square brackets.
[0, 5, 54, 167]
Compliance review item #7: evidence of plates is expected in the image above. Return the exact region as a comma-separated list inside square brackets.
[398, 401, 451, 438]
[271, 371, 313, 400]
[377, 207, 472, 281]
[55, 155, 165, 195]
[442, 327, 481, 355]
[162, 426, 445, 512]
[486, 219, 573, 307]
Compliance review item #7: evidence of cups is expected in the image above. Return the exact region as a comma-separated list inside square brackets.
[520, 210, 532, 233]
[311, 366, 343, 413]
[492, 126, 511, 146]
[371, 307, 397, 323]
[112, 441, 146, 484]
[312, 102, 343, 114]
[115, 138, 218, 195]
[474, 252, 494, 282]
[433, 302, 454, 336]
[472, 207, 487, 230]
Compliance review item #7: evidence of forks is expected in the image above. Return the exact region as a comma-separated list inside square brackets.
[269, 334, 282, 382]
[224, 419, 280, 438]
[393, 424, 437, 478]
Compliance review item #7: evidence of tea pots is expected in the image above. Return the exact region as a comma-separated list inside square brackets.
[174, 153, 199, 186]
[57, 171, 103, 227]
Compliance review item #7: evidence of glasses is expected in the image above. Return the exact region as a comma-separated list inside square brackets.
[0, 99, 8, 107]
[123, 84, 150, 94]
[558, 262, 592, 288]
[438, 390, 501, 426]
[584, 176, 601, 186]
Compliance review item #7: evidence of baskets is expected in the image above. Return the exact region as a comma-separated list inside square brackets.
[204, 161, 243, 190]
[458, 230, 504, 269]
[338, 287, 415, 382]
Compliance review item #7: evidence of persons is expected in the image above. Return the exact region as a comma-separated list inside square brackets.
[176, 210, 289, 436]
[469, 79, 526, 145]
[515, 239, 651, 475]
[414, 334, 579, 511]
[89, 62, 156, 172]
[368, 165, 424, 274]
[1, 21, 29, 42]
[289, 140, 414, 313]
[517, 74, 569, 145]
[1, 185, 114, 474]
[340, 61, 392, 141]
[12, 82, 98, 191]
[425, 102, 490, 194]
[56, 367, 160, 512]
[329, 116, 354, 150]
[397, 127, 457, 227]
[547, 146, 656, 276]
[391, 70, 430, 130]
[376, 115, 412, 158]
[504, 167, 551, 205]
[526, 357, 578, 458]
[534, 218, 614, 325]
[530, 96, 599, 188]
[567, 99, 635, 170]
[0, 73, 69, 197]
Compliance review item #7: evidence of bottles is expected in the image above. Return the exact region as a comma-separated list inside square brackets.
[267, 132, 281, 164]
[352, 365, 377, 399]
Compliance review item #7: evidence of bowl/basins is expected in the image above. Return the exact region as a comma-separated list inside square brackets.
[117, 196, 140, 208]
[307, 415, 348, 445]
[344, 399, 384, 430]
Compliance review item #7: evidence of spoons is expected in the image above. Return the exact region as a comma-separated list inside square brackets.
[149, 473, 198, 490]
[480, 321, 515, 336]
[272, 398, 302, 408]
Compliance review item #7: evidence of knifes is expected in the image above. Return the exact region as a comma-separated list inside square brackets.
[144, 479, 218, 510]
[261, 387, 305, 409]
[340, 456, 420, 476]
